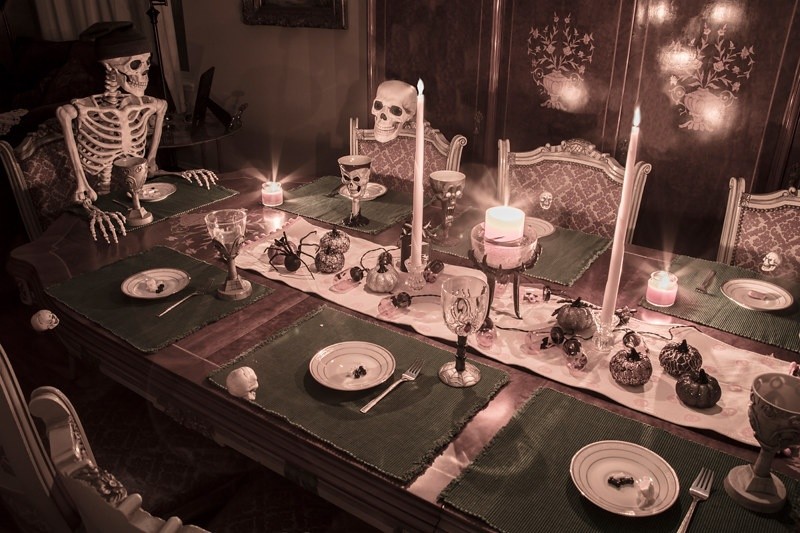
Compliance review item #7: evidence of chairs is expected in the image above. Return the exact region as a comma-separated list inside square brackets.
[499, 133, 650, 245]
[348, 114, 473, 193]
[25, 379, 213, 533]
[0, 120, 102, 244]
[715, 176, 800, 280]
[0, 344, 84, 532]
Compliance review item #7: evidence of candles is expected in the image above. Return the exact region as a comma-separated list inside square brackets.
[411, 79, 428, 273]
[482, 207, 526, 242]
[598, 106, 643, 329]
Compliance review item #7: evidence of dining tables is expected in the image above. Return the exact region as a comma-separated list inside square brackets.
[13, 169, 800, 533]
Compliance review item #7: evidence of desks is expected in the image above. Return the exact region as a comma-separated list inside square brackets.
[142, 106, 246, 167]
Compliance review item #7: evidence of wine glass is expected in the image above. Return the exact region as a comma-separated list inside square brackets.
[723, 373, 800, 514]
[338, 155, 371, 227]
[429, 170, 465, 247]
[113, 157, 154, 226]
[438, 276, 489, 388]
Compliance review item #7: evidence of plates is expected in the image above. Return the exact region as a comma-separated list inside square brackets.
[569, 440, 679, 516]
[309, 341, 396, 390]
[120, 267, 191, 299]
[339, 182, 387, 201]
[720, 277, 794, 311]
[524, 217, 556, 238]
[204, 209, 252, 300]
[127, 183, 177, 202]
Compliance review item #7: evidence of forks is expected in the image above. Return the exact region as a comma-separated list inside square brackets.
[155, 276, 216, 317]
[677, 466, 716, 533]
[360, 356, 425, 414]
[113, 199, 133, 211]
[695, 270, 717, 294]
[322, 183, 344, 197]
